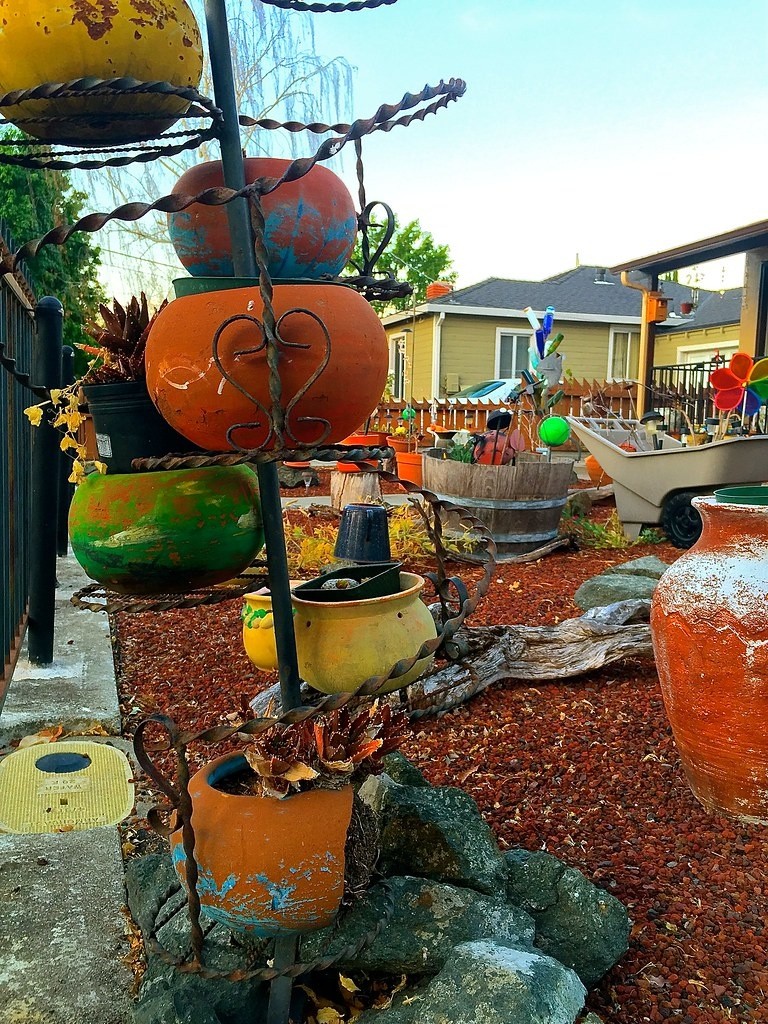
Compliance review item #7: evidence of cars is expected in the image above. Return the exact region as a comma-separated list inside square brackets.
[415, 377, 567, 419]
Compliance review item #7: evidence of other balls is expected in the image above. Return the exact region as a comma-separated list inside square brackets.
[403, 408, 416, 421]
[538, 416, 571, 447]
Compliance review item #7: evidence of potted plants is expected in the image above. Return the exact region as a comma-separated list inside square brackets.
[168, 702, 415, 937]
[70, 293, 222, 475]
[680, 301, 694, 314]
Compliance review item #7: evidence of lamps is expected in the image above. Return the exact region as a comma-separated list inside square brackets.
[704, 417, 720, 444]
[642, 410, 667, 450]
[679, 428, 690, 447]
[731, 419, 741, 436]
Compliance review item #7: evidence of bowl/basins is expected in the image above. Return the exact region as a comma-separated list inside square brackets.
[486, 410, 512, 430]
[437, 432, 458, 439]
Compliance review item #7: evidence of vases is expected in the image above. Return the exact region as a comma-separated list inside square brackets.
[68, 463, 438, 695]
[144, 157, 390, 451]
[338, 430, 422, 489]
[0, 1, 204, 148]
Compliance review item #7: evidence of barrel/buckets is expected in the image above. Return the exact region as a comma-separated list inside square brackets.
[331, 503, 392, 564]
